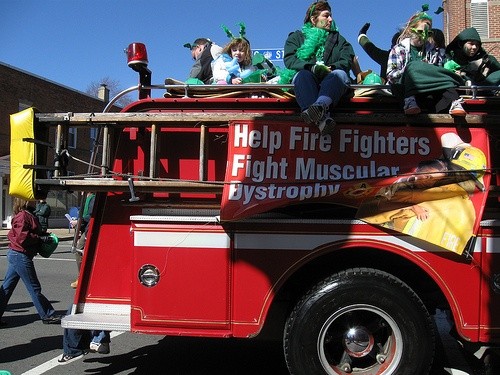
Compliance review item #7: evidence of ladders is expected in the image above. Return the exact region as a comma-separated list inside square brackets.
[8, 106, 500, 192]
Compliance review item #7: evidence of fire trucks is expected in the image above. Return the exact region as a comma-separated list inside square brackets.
[7, 41, 500, 375]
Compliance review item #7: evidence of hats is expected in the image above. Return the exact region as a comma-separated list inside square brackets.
[450, 143, 486, 192]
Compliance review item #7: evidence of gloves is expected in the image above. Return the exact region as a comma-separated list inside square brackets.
[358, 22, 370, 36]
[311, 64, 331, 80]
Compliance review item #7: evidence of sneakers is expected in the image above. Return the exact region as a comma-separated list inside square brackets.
[42, 312, 66, 324]
[404, 97, 421, 114]
[301, 103, 325, 125]
[319, 117, 336, 136]
[449, 98, 466, 117]
[57, 353, 88, 365]
[70, 280, 78, 288]
[90, 342, 110, 354]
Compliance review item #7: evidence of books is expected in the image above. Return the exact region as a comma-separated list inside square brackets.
[64, 207, 79, 228]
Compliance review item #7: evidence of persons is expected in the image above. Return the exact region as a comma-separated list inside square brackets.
[210, 36, 277, 84]
[183, 38, 214, 85]
[284, 0, 354, 134]
[444, 27, 500, 95]
[357, 13, 466, 117]
[55, 191, 113, 366]
[0, 196, 66, 325]
